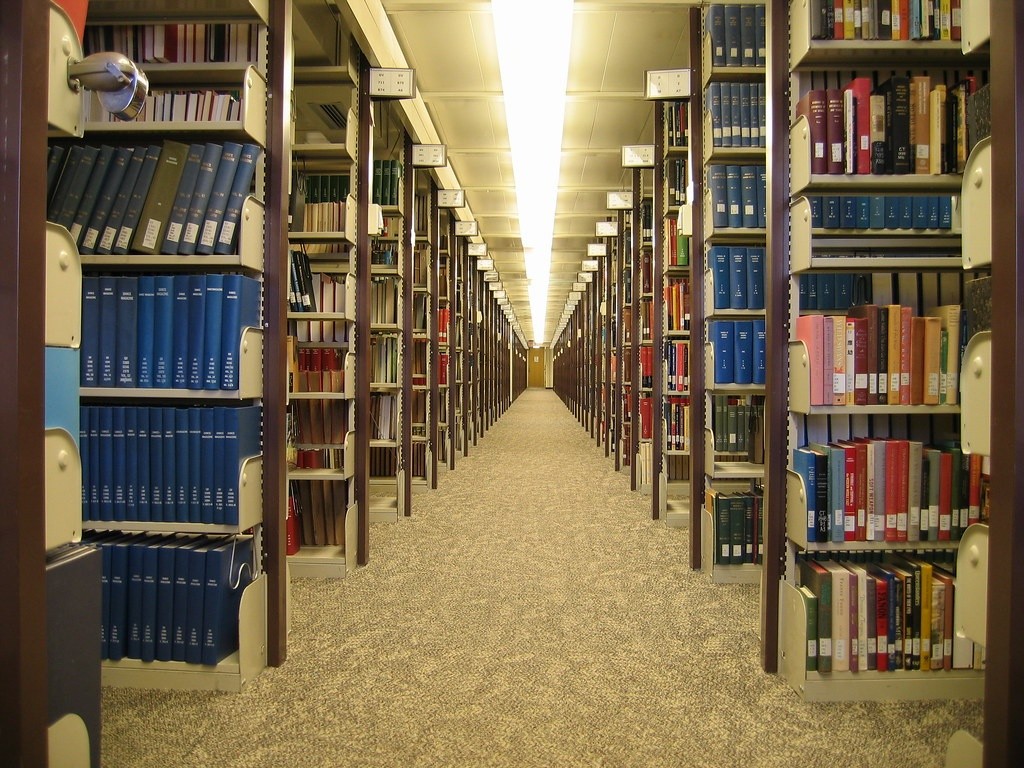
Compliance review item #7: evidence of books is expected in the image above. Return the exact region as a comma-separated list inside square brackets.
[576, 5, 766, 564]
[285, 157, 482, 556]
[791, 1, 993, 674]
[46, 23, 263, 668]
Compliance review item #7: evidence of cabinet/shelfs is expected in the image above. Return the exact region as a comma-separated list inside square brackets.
[0, 0, 1024, 768]
